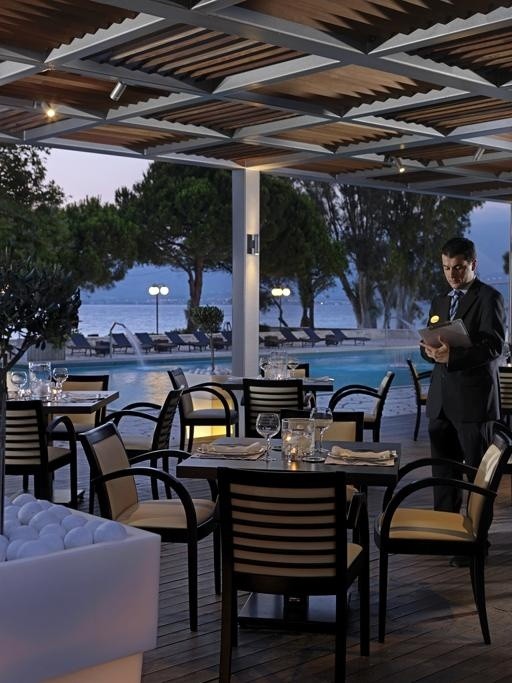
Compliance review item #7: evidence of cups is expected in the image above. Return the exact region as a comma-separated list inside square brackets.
[27, 363, 52, 399]
[284, 420, 314, 464]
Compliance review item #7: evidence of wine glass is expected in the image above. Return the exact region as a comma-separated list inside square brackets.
[255, 412, 281, 462]
[258, 356, 272, 380]
[287, 355, 300, 380]
[10, 372, 28, 399]
[309, 406, 333, 453]
[52, 367, 68, 400]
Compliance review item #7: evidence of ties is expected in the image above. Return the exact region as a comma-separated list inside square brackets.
[449, 291, 463, 320]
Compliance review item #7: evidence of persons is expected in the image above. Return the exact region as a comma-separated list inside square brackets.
[419, 238, 506, 568]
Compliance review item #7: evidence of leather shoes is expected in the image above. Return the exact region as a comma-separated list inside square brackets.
[450, 549, 488, 566]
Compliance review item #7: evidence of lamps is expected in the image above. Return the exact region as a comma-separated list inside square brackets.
[40, 102, 55, 118]
[111, 82, 125, 102]
[248, 233, 259, 256]
[391, 157, 407, 174]
[473, 145, 486, 164]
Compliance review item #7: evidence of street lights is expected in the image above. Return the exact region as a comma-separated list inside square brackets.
[147, 282, 170, 335]
[271, 286, 291, 326]
